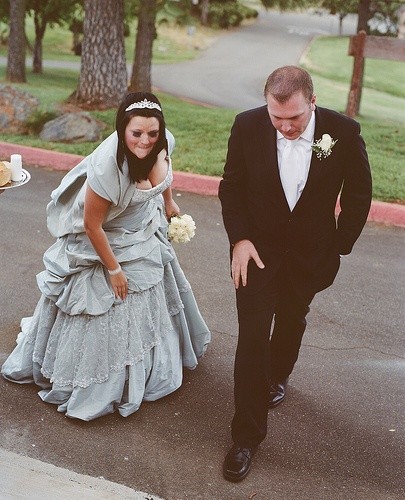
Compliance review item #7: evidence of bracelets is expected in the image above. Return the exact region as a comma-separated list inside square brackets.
[108, 263, 122, 276]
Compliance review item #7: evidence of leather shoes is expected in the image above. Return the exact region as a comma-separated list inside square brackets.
[267, 376, 289, 407]
[223, 443, 259, 482]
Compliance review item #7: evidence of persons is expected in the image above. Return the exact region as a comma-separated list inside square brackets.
[217, 67, 373, 480]
[0, 90, 211, 423]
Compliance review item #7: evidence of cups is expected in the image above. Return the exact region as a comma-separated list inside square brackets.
[10, 154, 23, 182]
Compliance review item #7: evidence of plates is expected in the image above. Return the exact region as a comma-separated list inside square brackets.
[0, 165, 31, 189]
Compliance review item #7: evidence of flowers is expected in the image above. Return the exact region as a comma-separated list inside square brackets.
[311, 134, 338, 160]
[168, 213, 196, 245]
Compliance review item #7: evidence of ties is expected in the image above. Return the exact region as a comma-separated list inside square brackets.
[279, 136, 301, 211]
